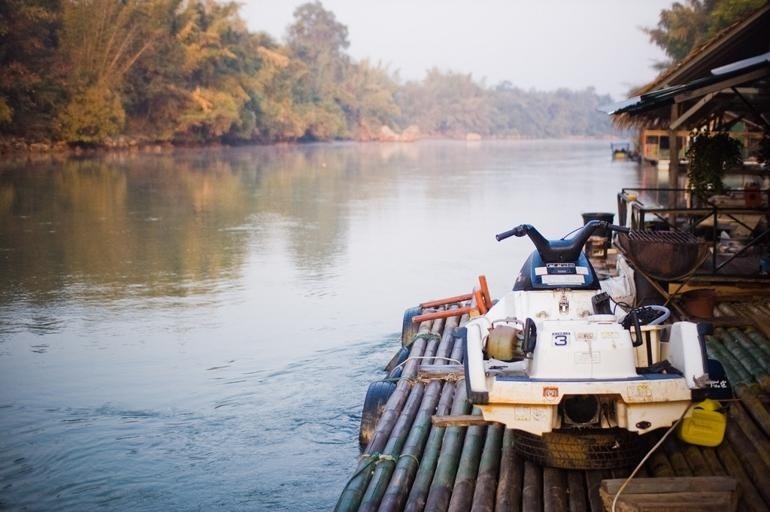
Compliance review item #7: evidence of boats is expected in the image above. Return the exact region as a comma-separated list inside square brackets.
[457, 216, 708, 438]
